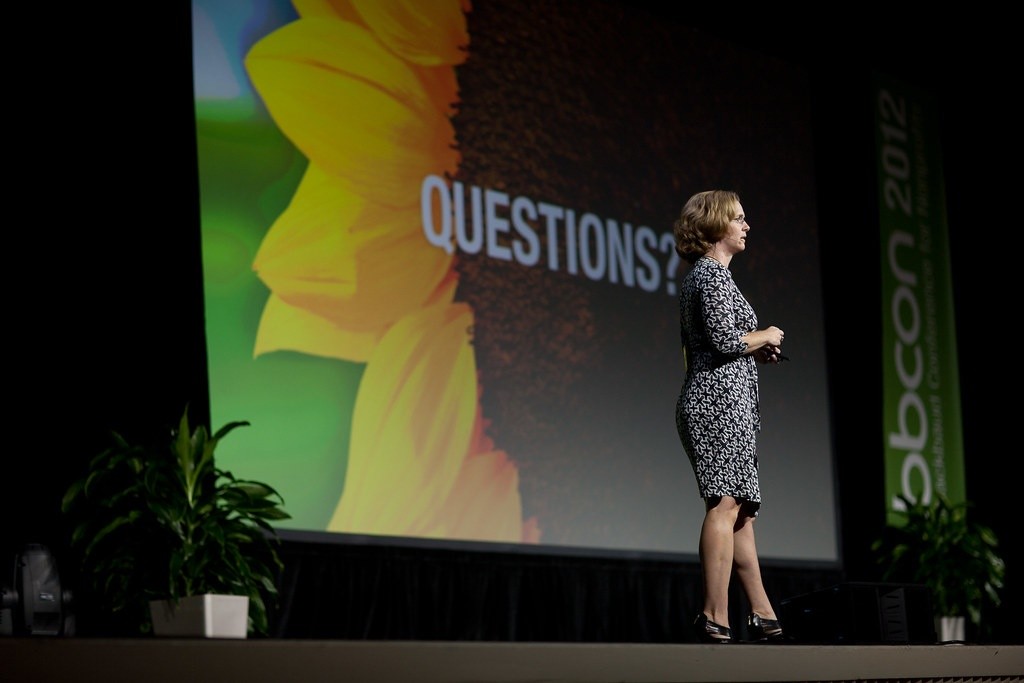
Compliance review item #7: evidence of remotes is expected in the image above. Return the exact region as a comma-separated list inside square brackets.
[763, 348, 791, 361]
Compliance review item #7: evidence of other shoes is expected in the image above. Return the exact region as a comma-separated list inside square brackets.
[691, 612, 737, 645]
[746, 612, 783, 644]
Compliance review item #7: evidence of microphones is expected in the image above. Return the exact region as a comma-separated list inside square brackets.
[726, 237, 742, 244]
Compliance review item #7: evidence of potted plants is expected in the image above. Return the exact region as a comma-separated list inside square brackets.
[871, 489, 1004, 646]
[61, 408, 292, 639]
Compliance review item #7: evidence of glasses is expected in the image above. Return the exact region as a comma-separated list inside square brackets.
[732, 215, 746, 224]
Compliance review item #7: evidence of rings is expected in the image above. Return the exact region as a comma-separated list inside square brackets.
[781, 336, 784, 341]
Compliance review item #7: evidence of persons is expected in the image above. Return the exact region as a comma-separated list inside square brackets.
[673, 190, 801, 644]
